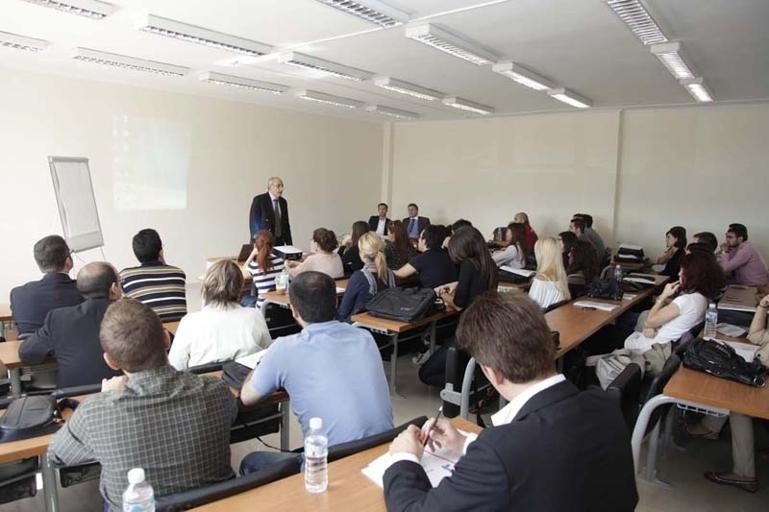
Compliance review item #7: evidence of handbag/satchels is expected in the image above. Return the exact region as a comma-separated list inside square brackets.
[596, 351, 646, 390]
[587, 266, 622, 300]
[221, 361, 251, 389]
[365, 288, 436, 323]
[682, 338, 766, 386]
[499, 270, 529, 283]
[0, 397, 58, 444]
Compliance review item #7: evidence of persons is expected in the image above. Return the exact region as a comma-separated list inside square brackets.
[17, 262, 174, 389]
[166, 261, 272, 372]
[239, 202, 500, 393]
[250, 177, 293, 249]
[566, 223, 768, 491]
[46, 298, 239, 511]
[489, 211, 606, 309]
[239, 271, 395, 477]
[383, 287, 639, 512]
[10, 235, 84, 337]
[113, 228, 185, 324]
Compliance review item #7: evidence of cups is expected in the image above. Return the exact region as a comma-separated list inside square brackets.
[275, 277, 287, 295]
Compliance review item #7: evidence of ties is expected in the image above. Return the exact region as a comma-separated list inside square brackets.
[273, 200, 282, 237]
[409, 219, 415, 232]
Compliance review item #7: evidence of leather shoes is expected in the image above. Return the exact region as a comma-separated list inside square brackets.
[705, 471, 759, 492]
[676, 420, 719, 440]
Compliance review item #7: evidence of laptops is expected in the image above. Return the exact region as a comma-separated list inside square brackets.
[232, 243, 254, 262]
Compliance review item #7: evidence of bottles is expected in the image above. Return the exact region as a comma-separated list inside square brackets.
[704, 303, 718, 338]
[120, 468, 156, 512]
[614, 265, 622, 282]
[303, 417, 329, 494]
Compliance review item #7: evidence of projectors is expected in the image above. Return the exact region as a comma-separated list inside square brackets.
[273, 244, 304, 262]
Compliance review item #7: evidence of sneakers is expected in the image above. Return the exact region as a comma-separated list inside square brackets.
[412, 349, 432, 364]
[470, 386, 496, 413]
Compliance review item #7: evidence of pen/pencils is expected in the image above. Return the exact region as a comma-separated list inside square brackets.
[423, 404, 444, 447]
